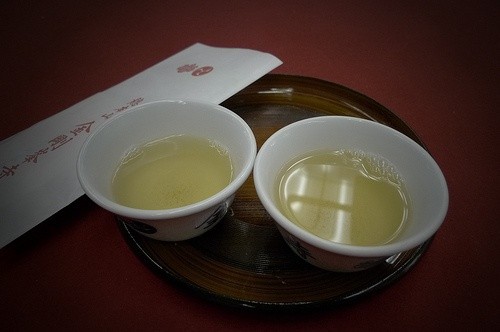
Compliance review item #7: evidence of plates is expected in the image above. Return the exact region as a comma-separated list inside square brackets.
[114, 74, 435, 315]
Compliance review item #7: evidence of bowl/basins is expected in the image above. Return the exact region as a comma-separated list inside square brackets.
[252, 115, 449, 273]
[76, 97, 257, 241]
